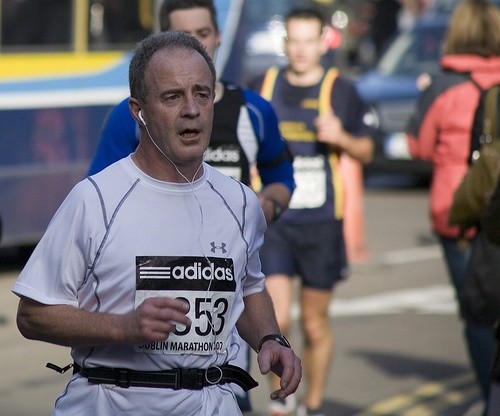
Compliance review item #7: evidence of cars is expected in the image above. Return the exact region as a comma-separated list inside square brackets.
[357, 13, 467, 186]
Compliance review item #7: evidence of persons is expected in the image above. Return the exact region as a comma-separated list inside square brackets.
[86, 0, 295, 220]
[255, 9, 379, 415]
[9, 30, 303, 416]
[407, 1, 499, 415]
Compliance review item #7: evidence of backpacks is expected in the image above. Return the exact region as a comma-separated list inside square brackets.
[466, 71, 500, 167]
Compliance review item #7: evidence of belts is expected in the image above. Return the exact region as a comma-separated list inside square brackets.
[72, 362, 261, 393]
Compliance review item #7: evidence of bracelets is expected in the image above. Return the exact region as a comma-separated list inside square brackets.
[270, 198, 282, 221]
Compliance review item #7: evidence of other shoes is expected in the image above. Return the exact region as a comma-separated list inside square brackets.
[267, 396, 300, 416]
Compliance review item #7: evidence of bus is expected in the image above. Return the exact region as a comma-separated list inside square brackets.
[0, 0, 347, 257]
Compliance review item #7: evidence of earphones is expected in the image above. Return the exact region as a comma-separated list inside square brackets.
[138, 109, 146, 126]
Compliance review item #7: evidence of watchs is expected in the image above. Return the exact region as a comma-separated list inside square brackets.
[256, 334, 292, 353]
